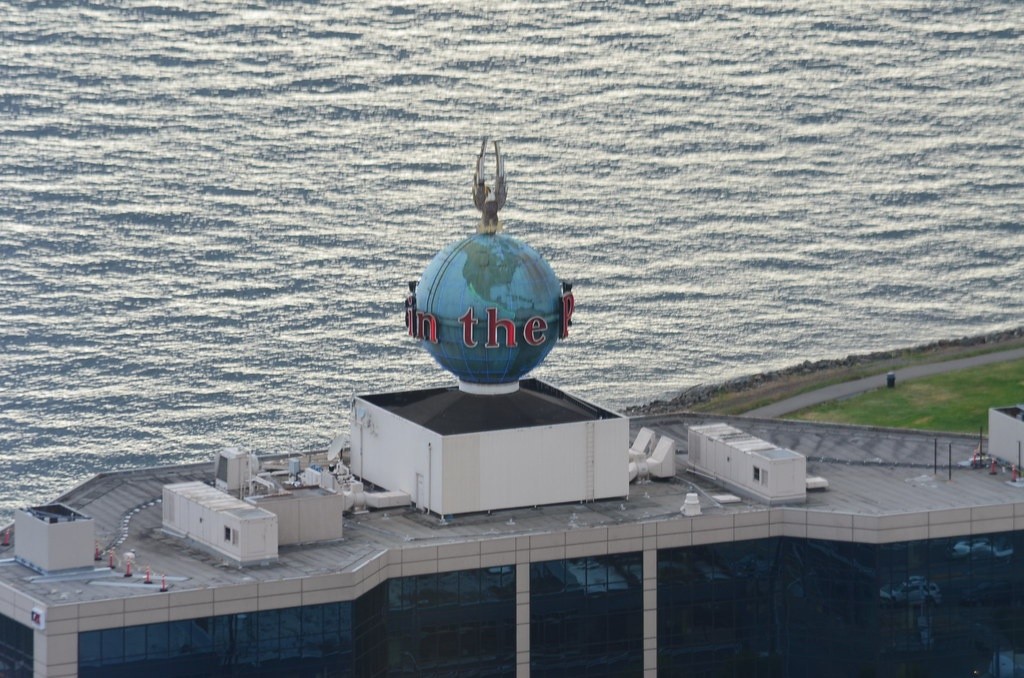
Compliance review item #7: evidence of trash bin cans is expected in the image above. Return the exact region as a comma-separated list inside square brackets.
[886, 371, 895, 387]
